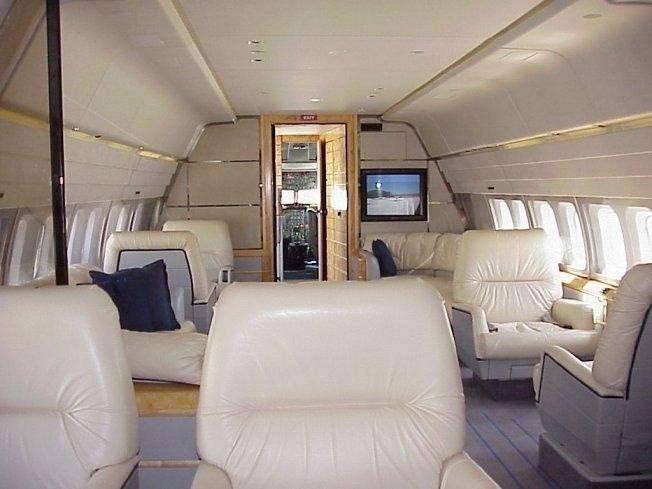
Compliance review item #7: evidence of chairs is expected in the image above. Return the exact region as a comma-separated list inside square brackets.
[532, 263, 652, 488]
[23, 219, 234, 414]
[190, 277, 502, 489]
[357, 231, 459, 315]
[451, 227, 607, 396]
[0, 284, 141, 489]
